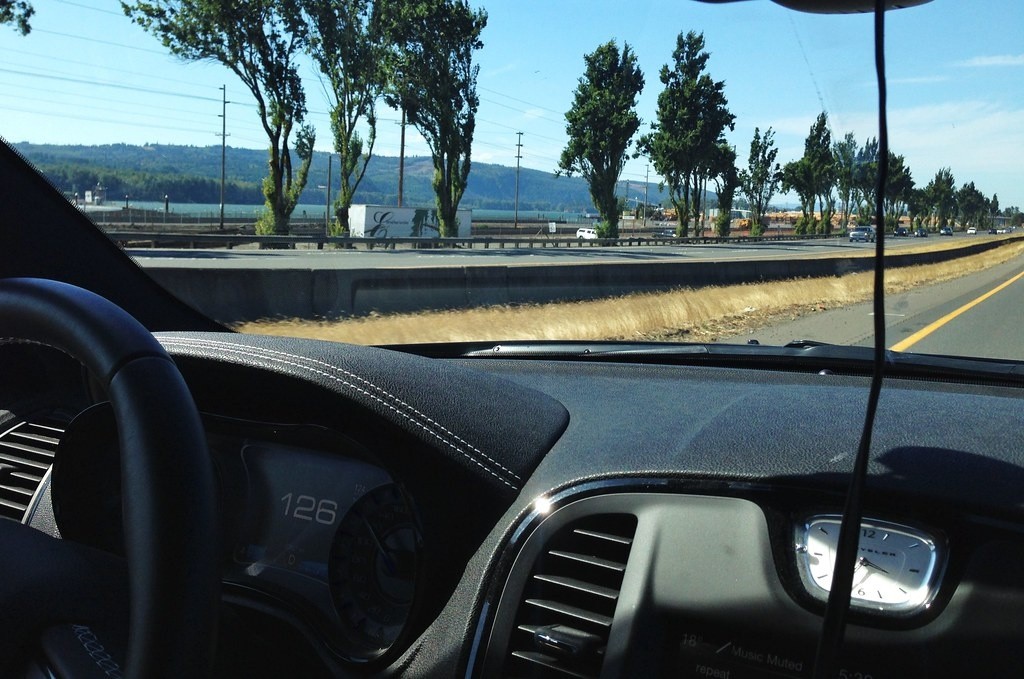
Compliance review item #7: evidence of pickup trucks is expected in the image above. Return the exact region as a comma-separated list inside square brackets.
[652, 229, 677, 242]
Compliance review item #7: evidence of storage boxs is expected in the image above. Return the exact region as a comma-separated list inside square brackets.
[348, 204, 472, 238]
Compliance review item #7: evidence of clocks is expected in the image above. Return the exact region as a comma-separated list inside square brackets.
[794, 510, 956, 624]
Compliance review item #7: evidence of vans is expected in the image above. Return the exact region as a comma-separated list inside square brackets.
[576, 228, 599, 240]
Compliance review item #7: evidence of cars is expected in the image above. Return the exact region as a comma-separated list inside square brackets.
[988, 228, 997, 234]
[893, 227, 909, 237]
[849, 225, 877, 243]
[940, 227, 954, 236]
[913, 228, 929, 237]
[966, 226, 977, 235]
[995, 224, 1018, 234]
